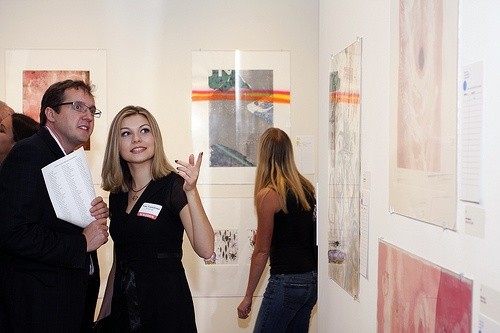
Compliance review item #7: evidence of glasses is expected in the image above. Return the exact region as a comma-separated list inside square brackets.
[51, 102, 102, 118]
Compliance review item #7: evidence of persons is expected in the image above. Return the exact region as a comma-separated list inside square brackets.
[92, 105, 215, 333]
[1, 79, 110, 333]
[236, 126, 318, 333]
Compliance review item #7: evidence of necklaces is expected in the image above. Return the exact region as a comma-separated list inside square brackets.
[130, 179, 153, 203]
